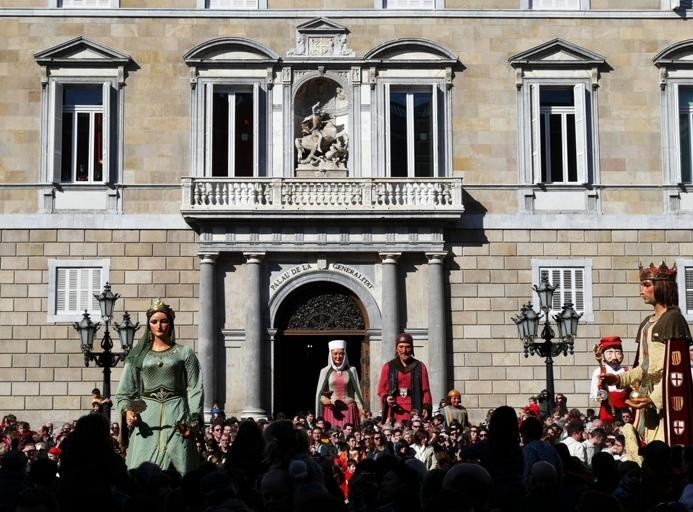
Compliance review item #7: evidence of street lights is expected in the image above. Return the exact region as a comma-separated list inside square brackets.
[511, 272, 584, 417]
[70, 282, 138, 426]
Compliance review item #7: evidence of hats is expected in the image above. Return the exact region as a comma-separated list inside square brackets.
[22, 442, 62, 456]
[638, 440, 671, 456]
[442, 464, 493, 489]
[532, 461, 557, 481]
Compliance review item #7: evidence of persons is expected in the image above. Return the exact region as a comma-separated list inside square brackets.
[0, 387, 693, 511]
[590, 260, 692, 464]
[375, 333, 431, 423]
[314, 339, 370, 427]
[112, 295, 204, 477]
[588, 336, 630, 422]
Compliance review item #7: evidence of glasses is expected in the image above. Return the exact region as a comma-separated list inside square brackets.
[331, 425, 420, 441]
[450, 430, 488, 438]
